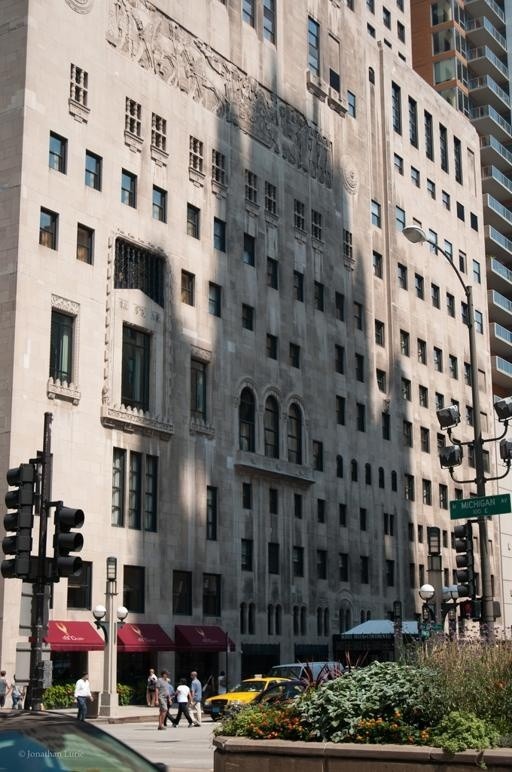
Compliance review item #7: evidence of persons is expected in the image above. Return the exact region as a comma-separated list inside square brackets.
[0, 670, 11, 709]
[172, 678, 195, 728]
[154, 669, 173, 730]
[189, 670, 202, 728]
[217, 671, 227, 695]
[146, 668, 157, 706]
[5, 673, 24, 710]
[163, 678, 178, 726]
[73, 672, 94, 722]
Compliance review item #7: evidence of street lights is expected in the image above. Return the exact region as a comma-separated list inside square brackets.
[418, 584, 435, 666]
[93, 556, 129, 716]
[402, 224, 512, 642]
[442, 584, 460, 652]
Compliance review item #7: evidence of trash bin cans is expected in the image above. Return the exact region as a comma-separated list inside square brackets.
[86, 692, 99, 719]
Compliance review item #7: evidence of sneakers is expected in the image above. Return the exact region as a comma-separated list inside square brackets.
[157, 722, 202, 731]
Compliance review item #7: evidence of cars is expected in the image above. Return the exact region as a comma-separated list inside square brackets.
[0, 708, 169, 772]
[203, 677, 302, 721]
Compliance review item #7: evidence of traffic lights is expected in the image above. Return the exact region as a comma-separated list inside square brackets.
[0, 459, 85, 583]
[460, 599, 482, 620]
[453, 520, 476, 598]
[492, 601, 502, 619]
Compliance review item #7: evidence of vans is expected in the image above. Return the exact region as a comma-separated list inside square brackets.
[272, 661, 345, 682]
